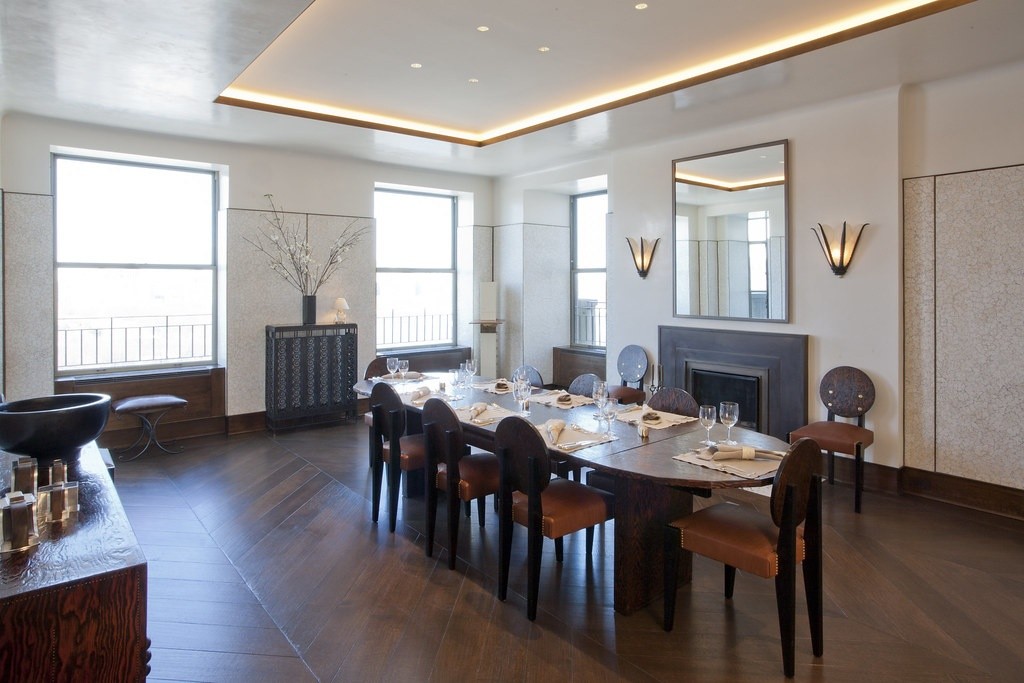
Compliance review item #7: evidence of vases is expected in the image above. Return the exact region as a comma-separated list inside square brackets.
[302, 293, 319, 323]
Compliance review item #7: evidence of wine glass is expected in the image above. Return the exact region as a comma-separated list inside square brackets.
[398, 360, 410, 385]
[449, 358, 477, 400]
[699, 405, 717, 446]
[513, 379, 532, 419]
[592, 381, 618, 440]
[719, 401, 739, 446]
[387, 358, 398, 385]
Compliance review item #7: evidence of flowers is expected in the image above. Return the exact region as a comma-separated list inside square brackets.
[241, 188, 372, 290]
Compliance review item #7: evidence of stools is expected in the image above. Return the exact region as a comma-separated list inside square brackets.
[104, 394, 189, 462]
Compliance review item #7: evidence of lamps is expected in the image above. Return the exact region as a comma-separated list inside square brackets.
[811, 220, 868, 275]
[333, 298, 349, 321]
[626, 236, 661, 277]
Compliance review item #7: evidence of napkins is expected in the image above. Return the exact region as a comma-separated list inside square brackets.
[469, 401, 487, 420]
[640, 404, 662, 425]
[696, 443, 781, 462]
[496, 378, 508, 391]
[543, 419, 566, 445]
[382, 372, 422, 380]
[557, 394, 584, 405]
[405, 387, 431, 401]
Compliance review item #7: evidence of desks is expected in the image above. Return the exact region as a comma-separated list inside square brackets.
[266, 322, 361, 435]
[0, 443, 151, 683]
[354, 371, 787, 613]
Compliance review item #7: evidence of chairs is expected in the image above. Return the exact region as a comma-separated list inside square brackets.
[661, 436, 828, 677]
[371, 383, 473, 530]
[571, 370, 607, 404]
[511, 363, 543, 388]
[586, 388, 699, 555]
[424, 398, 505, 569]
[496, 417, 615, 621]
[609, 345, 648, 407]
[791, 365, 878, 513]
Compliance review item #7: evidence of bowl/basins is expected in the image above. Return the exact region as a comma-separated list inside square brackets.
[0, 392, 112, 468]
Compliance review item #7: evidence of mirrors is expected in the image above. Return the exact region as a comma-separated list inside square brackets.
[670, 138, 789, 323]
